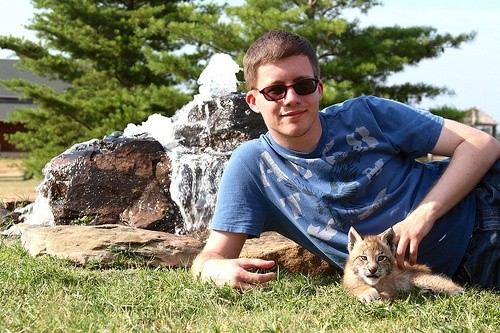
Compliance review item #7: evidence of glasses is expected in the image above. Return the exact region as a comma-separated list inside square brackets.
[255, 77, 319, 101]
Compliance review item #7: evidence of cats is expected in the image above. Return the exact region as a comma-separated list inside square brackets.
[342, 226, 465, 305]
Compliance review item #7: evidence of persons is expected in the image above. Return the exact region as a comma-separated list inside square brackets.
[188, 28, 500, 301]
